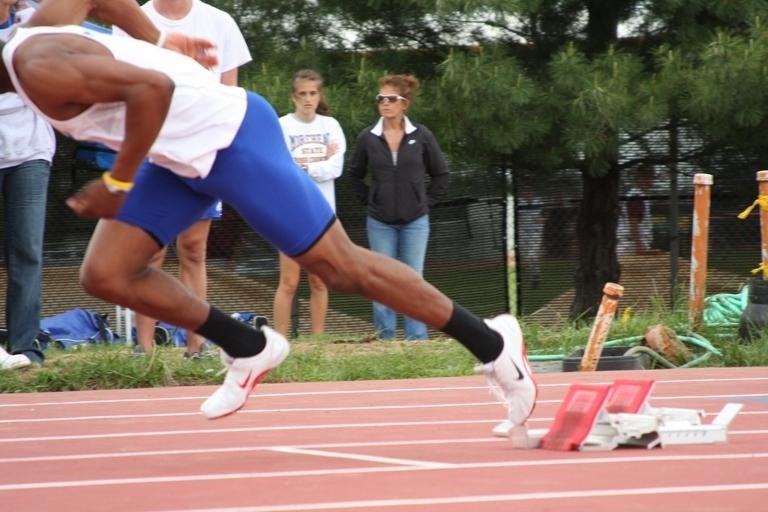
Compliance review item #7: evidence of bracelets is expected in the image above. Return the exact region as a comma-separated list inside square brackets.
[102, 171, 135, 193]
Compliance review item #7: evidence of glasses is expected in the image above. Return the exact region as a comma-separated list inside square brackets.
[375, 94, 409, 103]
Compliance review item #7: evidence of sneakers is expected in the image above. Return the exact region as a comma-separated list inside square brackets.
[200, 325, 290, 420]
[473, 314, 538, 438]
[0, 347, 44, 371]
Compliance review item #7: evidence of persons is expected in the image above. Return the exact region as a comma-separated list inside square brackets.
[0, 0, 539, 438]
[616, 164, 660, 258]
[0, 0, 59, 371]
[348, 74, 451, 339]
[93, 0, 254, 363]
[516, 184, 582, 261]
[271, 69, 348, 340]
